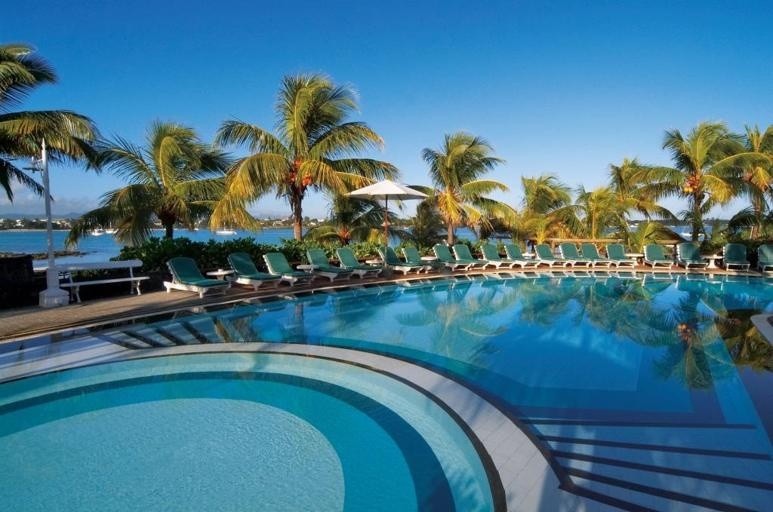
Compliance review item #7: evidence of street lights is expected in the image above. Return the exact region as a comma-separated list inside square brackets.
[21, 137, 70, 309]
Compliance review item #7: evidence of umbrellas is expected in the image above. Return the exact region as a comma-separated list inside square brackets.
[343, 179, 430, 269]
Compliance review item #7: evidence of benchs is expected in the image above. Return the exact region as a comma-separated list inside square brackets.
[55, 259, 151, 305]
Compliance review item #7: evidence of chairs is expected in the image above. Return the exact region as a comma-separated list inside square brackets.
[674, 242, 711, 272]
[376, 242, 641, 278]
[296, 248, 355, 284]
[261, 252, 317, 290]
[720, 243, 752, 273]
[162, 255, 231, 300]
[643, 244, 674, 272]
[333, 247, 383, 281]
[225, 251, 283, 294]
[755, 242, 773, 277]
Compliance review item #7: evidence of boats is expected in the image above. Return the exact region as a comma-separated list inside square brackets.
[89, 228, 116, 236]
[679, 231, 704, 238]
[215, 228, 235, 235]
